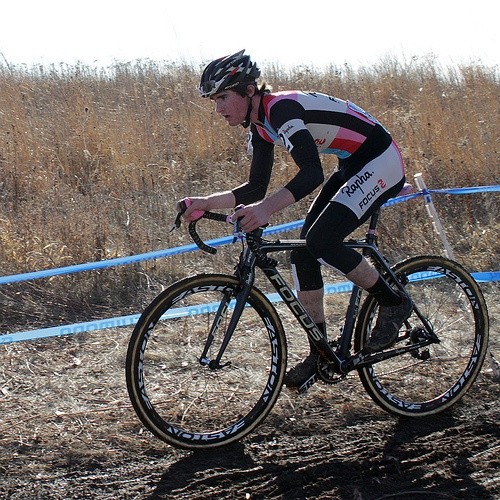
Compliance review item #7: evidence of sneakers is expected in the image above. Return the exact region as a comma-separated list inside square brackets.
[370, 290, 414, 346]
[284, 354, 327, 390]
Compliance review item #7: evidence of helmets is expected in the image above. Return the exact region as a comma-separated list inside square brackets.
[199, 49, 261, 98]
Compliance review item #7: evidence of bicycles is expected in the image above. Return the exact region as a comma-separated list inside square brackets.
[124, 183, 490, 452]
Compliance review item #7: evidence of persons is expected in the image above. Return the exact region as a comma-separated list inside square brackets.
[175, 48, 417, 387]
